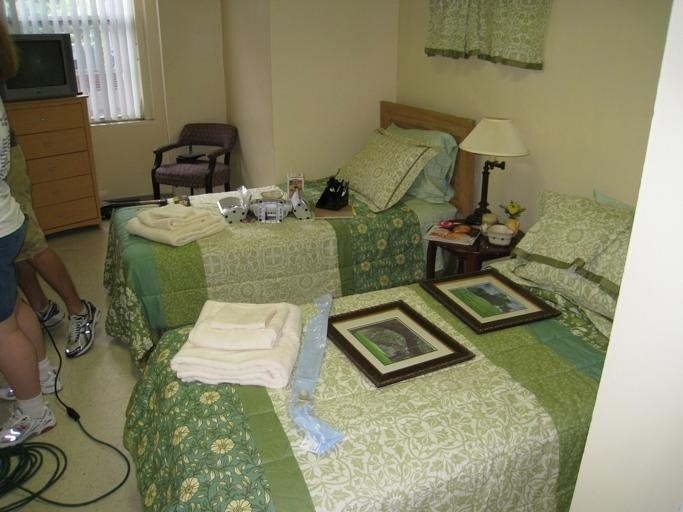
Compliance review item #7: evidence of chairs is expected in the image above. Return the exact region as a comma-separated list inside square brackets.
[151, 123, 237, 200]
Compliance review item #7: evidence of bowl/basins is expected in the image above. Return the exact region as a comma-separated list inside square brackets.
[487, 225, 513, 246]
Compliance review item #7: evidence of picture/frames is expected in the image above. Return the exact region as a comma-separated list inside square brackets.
[328, 299, 475, 387]
[418, 268, 562, 334]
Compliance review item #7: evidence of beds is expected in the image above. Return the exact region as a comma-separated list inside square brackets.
[123, 261, 616, 512]
[103, 100, 476, 371]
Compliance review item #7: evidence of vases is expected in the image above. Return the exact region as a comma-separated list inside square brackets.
[506, 219, 520, 238]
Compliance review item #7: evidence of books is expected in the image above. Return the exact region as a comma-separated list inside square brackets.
[425, 221, 482, 246]
[314, 205, 353, 219]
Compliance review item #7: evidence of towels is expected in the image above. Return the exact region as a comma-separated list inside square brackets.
[125, 209, 228, 247]
[187, 304, 291, 350]
[212, 306, 277, 329]
[149, 203, 194, 218]
[169, 298, 301, 389]
[138, 204, 214, 231]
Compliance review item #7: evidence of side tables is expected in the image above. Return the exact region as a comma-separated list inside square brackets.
[425, 221, 524, 282]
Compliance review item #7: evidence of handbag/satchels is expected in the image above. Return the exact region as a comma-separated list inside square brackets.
[315, 177, 347, 210]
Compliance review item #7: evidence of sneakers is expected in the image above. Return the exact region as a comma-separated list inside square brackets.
[0, 367, 62, 401]
[0, 402, 56, 449]
[35, 300, 64, 328]
[64, 299, 101, 358]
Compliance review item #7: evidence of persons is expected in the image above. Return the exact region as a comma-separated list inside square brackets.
[0, 100, 61, 448]
[0, 1, 101, 360]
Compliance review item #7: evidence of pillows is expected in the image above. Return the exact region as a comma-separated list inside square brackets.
[484, 259, 614, 352]
[334, 129, 440, 214]
[512, 189, 635, 320]
[382, 120, 458, 206]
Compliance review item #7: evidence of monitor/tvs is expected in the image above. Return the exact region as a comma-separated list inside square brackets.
[1, 34, 76, 104]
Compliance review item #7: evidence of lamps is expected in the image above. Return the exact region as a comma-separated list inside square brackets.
[458, 117, 529, 224]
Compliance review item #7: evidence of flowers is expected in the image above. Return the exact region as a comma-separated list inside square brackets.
[500, 200, 525, 218]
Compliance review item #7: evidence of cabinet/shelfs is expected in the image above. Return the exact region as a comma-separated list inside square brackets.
[4, 95, 102, 240]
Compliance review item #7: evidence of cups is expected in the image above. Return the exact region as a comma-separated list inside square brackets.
[480, 214, 496, 237]
